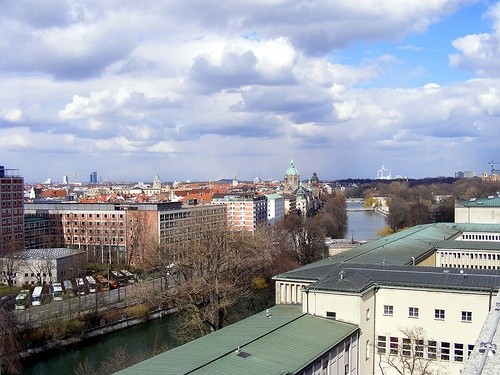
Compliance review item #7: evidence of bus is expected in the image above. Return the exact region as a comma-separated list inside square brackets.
[64, 280, 75, 298]
[111, 270, 126, 288]
[85, 276, 98, 294]
[15, 290, 31, 311]
[31, 286, 45, 307]
[76, 278, 86, 296]
[120, 270, 135, 286]
[164, 261, 178, 275]
[96, 274, 110, 294]
[52, 282, 64, 302]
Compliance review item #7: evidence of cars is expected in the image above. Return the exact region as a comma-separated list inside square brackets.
[325, 239, 337, 244]
